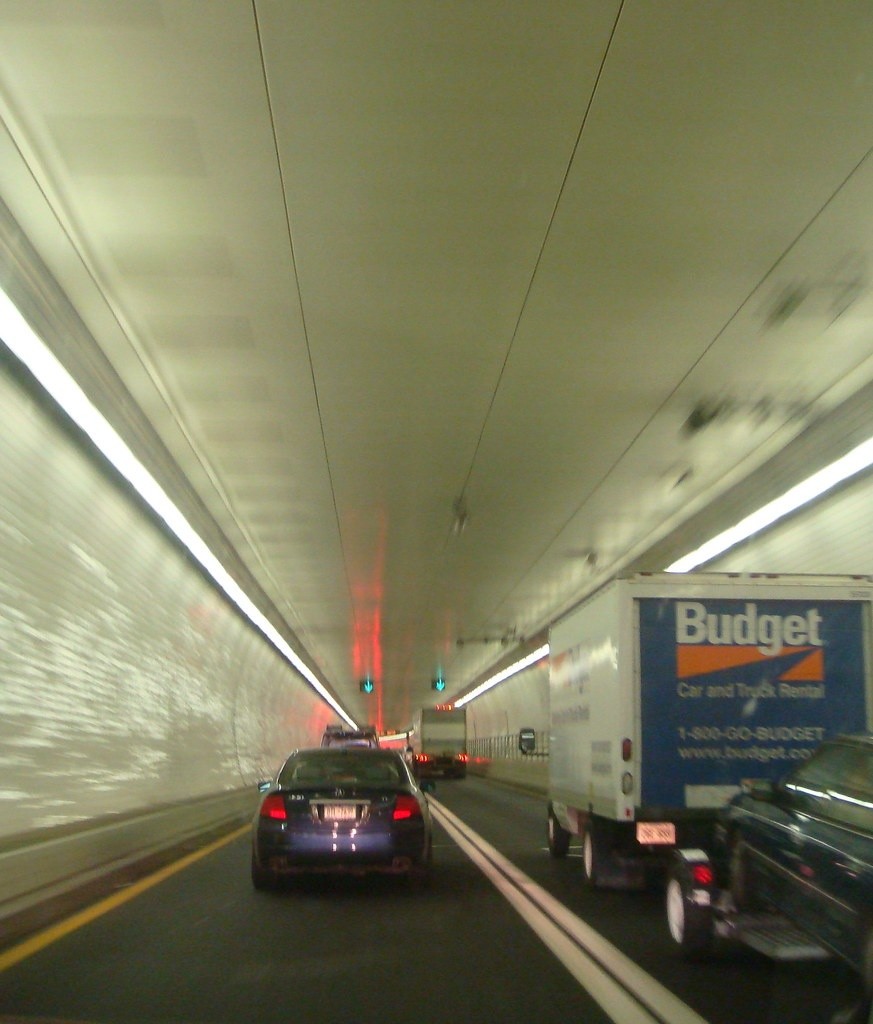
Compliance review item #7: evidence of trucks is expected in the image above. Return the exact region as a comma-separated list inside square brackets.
[519, 572, 873, 898]
[412, 708, 466, 779]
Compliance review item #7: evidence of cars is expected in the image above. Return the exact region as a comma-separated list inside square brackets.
[250, 749, 433, 892]
[663, 730, 873, 984]
